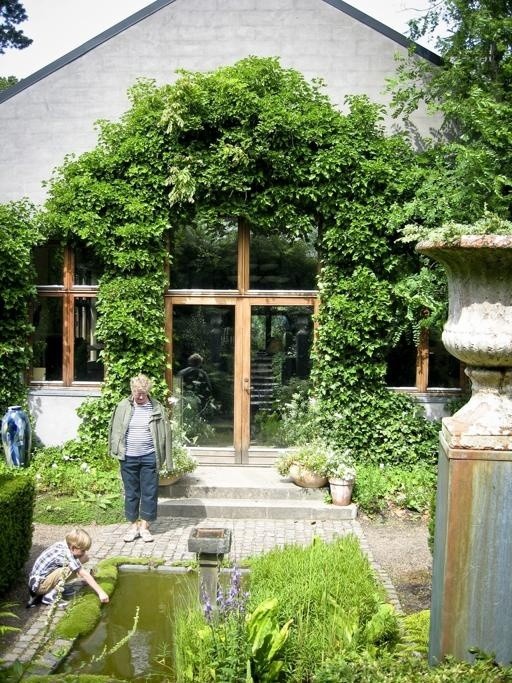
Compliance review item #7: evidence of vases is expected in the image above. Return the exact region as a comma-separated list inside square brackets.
[1, 406, 32, 468]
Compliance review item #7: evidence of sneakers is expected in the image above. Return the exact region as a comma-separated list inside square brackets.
[140, 527, 154, 542]
[42, 594, 69, 607]
[123, 528, 139, 541]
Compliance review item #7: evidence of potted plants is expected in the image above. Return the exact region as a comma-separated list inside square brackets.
[393, 199, 512, 452]
[277, 441, 329, 488]
[158, 447, 195, 486]
[326, 466, 356, 506]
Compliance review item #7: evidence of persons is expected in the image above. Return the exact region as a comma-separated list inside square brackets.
[29, 527, 114, 607]
[108, 373, 171, 543]
[174, 351, 214, 448]
